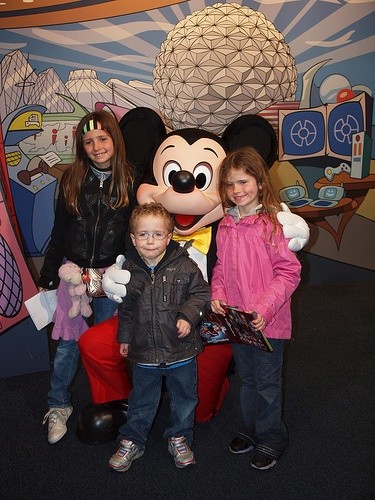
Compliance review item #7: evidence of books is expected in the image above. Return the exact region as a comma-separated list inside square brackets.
[192, 306, 274, 353]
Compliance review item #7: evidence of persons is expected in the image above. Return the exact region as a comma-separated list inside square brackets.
[40, 108, 141, 442]
[82, 106, 310, 447]
[207, 147, 309, 470]
[109, 204, 207, 471]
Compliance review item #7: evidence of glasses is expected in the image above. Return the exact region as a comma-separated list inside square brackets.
[132, 231, 168, 241]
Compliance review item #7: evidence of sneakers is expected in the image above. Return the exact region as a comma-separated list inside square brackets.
[251, 450, 277, 469]
[108, 439, 144, 472]
[43, 406, 73, 444]
[168, 436, 196, 468]
[229, 437, 254, 453]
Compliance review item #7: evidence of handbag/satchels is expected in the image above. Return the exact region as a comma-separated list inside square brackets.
[85, 268, 107, 297]
